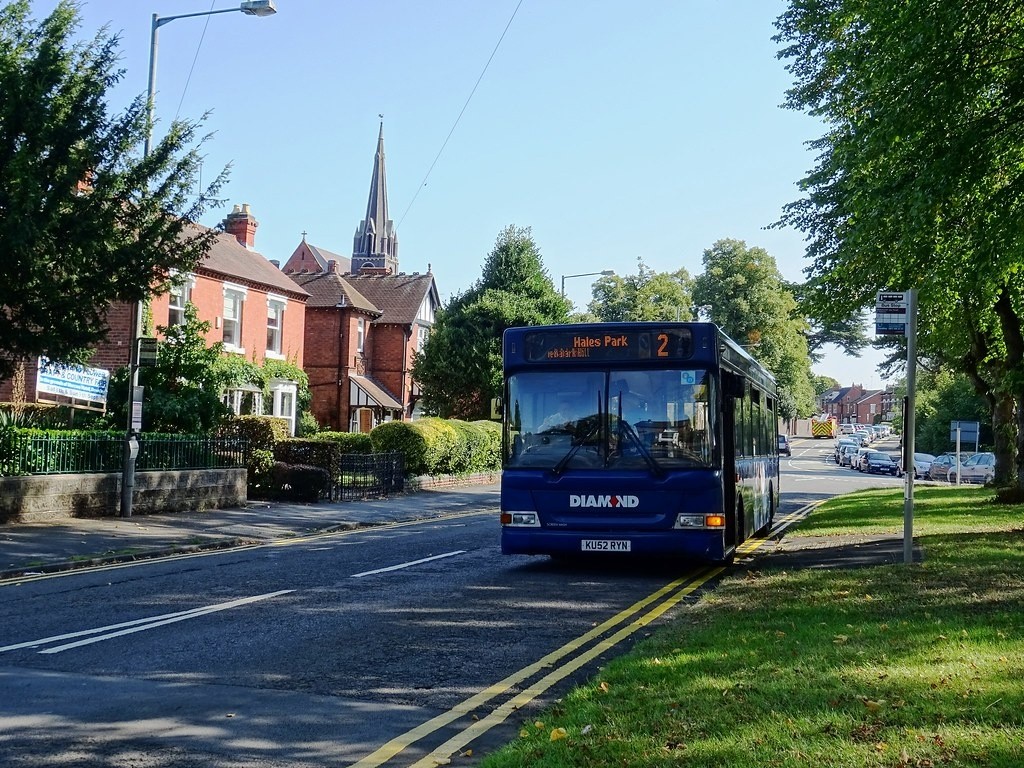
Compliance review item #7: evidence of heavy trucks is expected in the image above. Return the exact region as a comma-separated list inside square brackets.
[811, 414, 838, 439]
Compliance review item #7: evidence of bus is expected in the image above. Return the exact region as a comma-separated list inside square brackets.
[500, 322, 780, 569]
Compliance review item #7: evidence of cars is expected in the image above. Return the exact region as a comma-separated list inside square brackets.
[777, 433, 792, 456]
[946, 453, 996, 484]
[832, 421, 896, 476]
[895, 453, 936, 480]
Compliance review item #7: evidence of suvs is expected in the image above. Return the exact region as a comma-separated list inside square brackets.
[927, 452, 969, 482]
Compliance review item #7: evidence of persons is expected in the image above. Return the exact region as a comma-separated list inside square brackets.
[537, 400, 578, 434]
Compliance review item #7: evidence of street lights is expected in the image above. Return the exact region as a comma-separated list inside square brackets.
[120, 1, 278, 519]
[561, 269, 615, 298]
